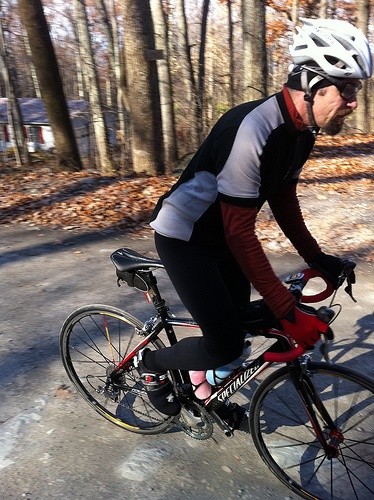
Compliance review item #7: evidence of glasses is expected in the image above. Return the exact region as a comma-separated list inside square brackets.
[297, 65, 363, 100]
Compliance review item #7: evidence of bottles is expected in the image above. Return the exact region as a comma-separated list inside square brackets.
[206, 340, 252, 385]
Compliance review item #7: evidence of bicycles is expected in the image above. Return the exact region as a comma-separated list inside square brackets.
[59, 247, 374, 500]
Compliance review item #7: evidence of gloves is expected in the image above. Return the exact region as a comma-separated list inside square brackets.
[311, 255, 356, 286]
[282, 302, 336, 350]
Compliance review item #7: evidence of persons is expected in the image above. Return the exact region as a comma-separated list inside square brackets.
[131, 17, 374, 430]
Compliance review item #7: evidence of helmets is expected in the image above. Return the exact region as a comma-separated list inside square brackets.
[288, 16, 373, 79]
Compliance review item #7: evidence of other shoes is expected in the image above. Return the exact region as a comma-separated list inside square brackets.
[219, 399, 248, 424]
[132, 348, 181, 419]
